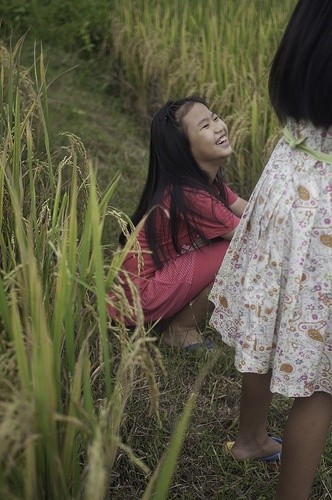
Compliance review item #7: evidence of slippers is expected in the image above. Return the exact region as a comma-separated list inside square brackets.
[222, 437, 284, 461]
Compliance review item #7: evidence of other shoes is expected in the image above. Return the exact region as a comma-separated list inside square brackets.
[158, 334, 214, 362]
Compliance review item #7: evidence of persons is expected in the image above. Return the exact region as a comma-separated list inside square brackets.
[99, 95, 251, 363]
[209, 0, 331, 500]
